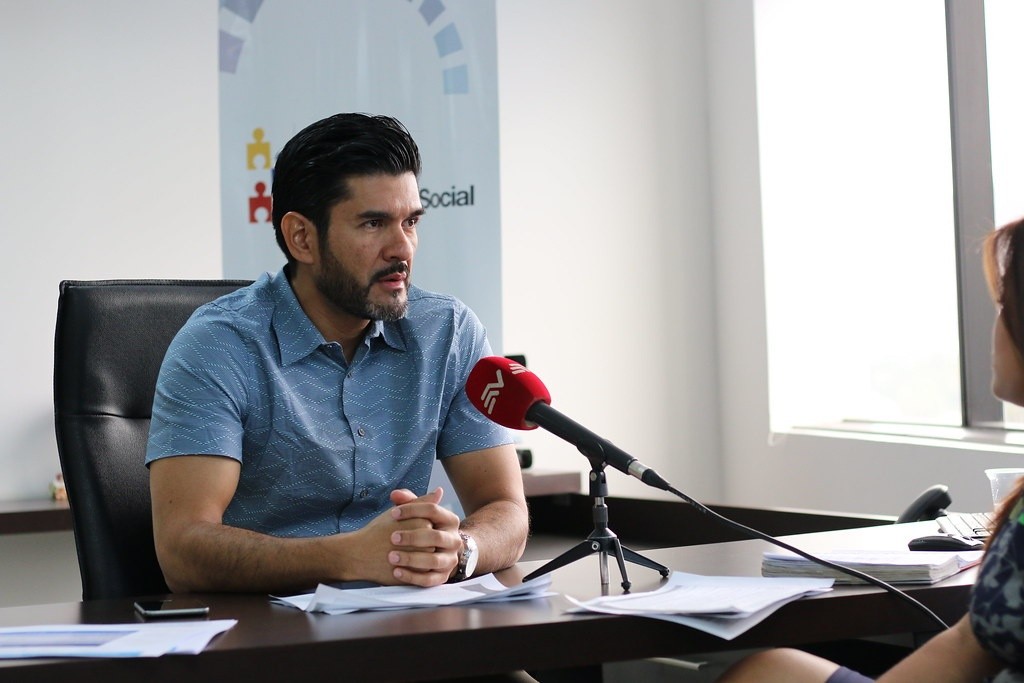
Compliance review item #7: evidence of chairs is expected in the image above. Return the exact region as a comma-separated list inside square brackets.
[47, 274, 283, 626]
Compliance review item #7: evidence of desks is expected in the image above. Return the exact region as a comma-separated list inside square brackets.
[0, 508, 1008, 682]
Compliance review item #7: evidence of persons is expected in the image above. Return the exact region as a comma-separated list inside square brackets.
[716, 216, 1024, 683]
[145, 112, 528, 594]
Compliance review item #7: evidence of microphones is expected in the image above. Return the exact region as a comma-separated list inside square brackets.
[465, 356, 670, 491]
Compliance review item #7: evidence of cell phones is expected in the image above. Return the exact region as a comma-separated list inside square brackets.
[134, 597, 210, 617]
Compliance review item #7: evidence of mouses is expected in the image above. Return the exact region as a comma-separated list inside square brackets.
[908, 534, 984, 551]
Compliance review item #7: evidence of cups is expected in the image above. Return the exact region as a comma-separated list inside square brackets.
[984, 466, 1024, 512]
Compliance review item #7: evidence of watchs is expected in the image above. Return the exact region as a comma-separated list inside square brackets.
[451, 530, 480, 582]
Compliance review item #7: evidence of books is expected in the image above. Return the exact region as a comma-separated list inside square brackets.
[762, 550, 985, 585]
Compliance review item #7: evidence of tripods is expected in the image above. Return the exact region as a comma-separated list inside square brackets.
[522, 467, 669, 599]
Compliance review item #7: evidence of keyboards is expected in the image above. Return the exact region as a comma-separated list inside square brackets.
[935, 512, 1000, 542]
